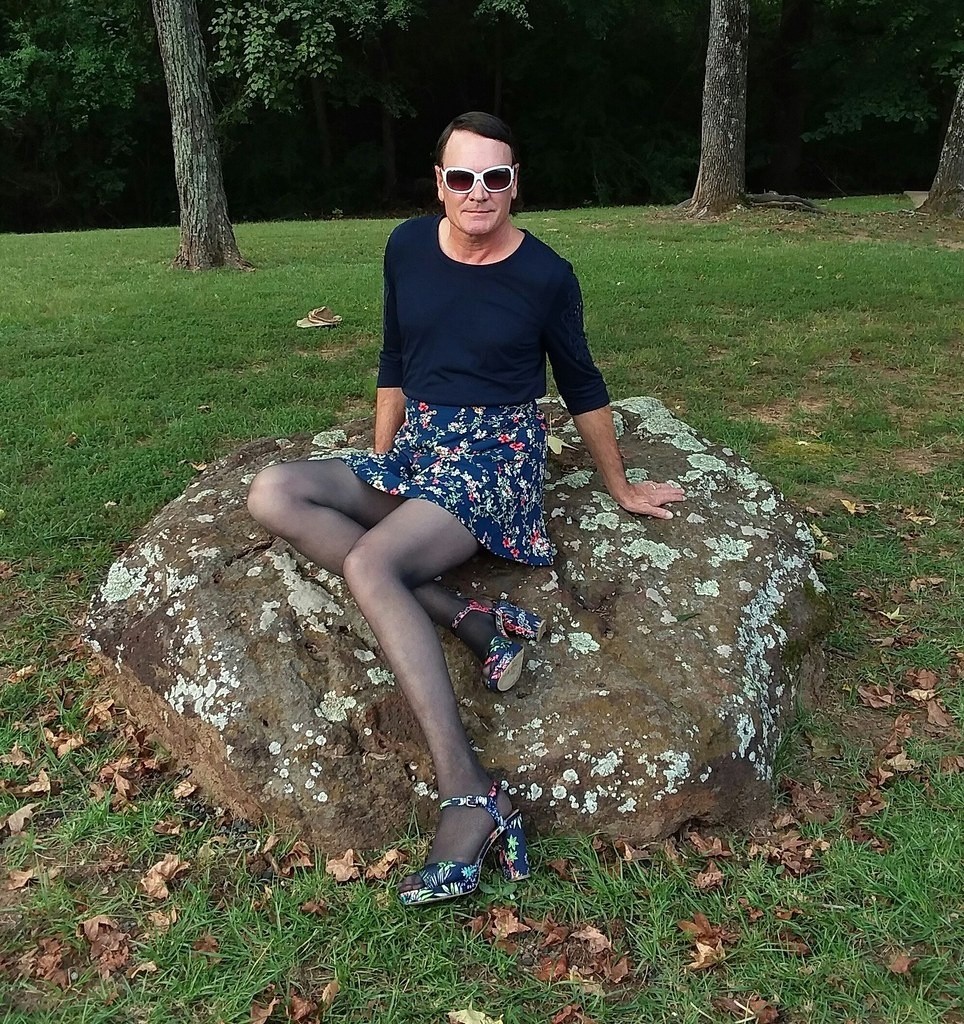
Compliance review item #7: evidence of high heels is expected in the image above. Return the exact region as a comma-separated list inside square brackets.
[448, 599, 546, 689]
[397, 780, 530, 905]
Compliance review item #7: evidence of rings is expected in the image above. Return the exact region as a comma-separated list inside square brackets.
[649, 483, 657, 491]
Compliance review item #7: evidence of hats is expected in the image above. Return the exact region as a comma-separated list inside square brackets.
[297, 305, 342, 328]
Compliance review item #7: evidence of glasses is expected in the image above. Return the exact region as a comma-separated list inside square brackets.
[439, 165, 516, 194]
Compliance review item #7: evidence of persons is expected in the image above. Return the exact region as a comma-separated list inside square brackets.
[244, 110, 685, 906]
